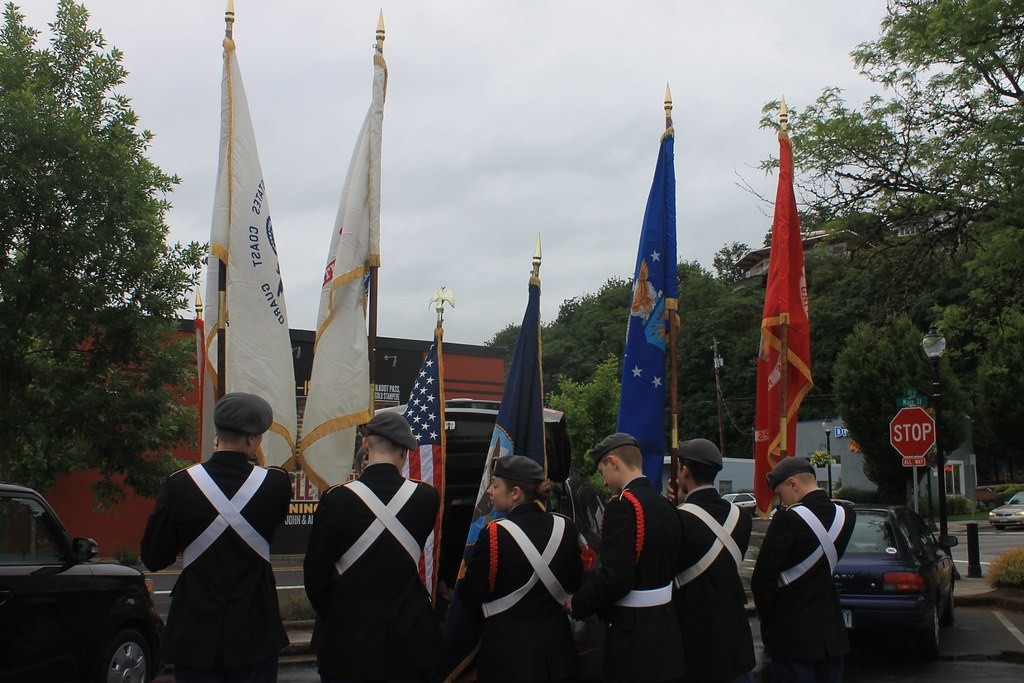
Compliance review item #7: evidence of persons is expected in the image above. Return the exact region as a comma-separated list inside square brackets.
[462, 455, 584, 683]
[304, 412, 441, 683]
[140, 393, 292, 683]
[666, 438, 757, 683]
[567, 433, 682, 683]
[751, 457, 856, 683]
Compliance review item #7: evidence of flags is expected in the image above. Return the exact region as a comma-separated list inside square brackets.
[204, 40, 298, 468]
[301, 49, 381, 492]
[754, 131, 813, 520]
[458, 273, 546, 599]
[615, 126, 680, 495]
[195, 318, 220, 464]
[401, 324, 442, 597]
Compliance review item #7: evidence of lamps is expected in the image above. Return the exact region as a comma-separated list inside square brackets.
[291, 346, 301, 358]
[384, 356, 397, 367]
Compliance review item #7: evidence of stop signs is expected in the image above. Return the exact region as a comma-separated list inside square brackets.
[890, 406, 936, 459]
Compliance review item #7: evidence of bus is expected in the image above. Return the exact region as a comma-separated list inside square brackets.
[366, 398, 567, 597]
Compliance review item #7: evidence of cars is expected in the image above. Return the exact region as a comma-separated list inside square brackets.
[0, 484, 171, 683]
[720, 493, 759, 516]
[833, 504, 960, 652]
[989, 490, 1024, 531]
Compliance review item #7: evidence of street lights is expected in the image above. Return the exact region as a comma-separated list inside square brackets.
[822, 416, 834, 500]
[922, 321, 951, 566]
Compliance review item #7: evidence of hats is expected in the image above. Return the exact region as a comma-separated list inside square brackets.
[766, 455, 816, 489]
[673, 438, 724, 469]
[587, 433, 640, 468]
[493, 454, 546, 482]
[214, 392, 273, 435]
[366, 412, 417, 450]
